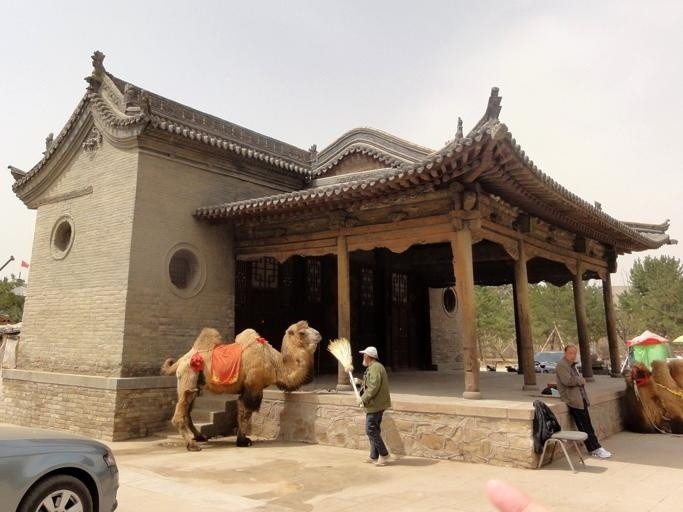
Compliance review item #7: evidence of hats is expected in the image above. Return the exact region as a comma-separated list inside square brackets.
[359, 347, 378, 358]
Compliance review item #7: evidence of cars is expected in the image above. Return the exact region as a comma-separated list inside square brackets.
[0, 425, 119, 512]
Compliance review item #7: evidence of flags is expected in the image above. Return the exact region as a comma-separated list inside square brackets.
[21, 260, 29, 269]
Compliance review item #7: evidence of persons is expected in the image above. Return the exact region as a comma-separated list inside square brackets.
[349, 345, 393, 466]
[556, 344, 612, 459]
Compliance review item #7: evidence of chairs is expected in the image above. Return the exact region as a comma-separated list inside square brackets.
[533, 400, 589, 474]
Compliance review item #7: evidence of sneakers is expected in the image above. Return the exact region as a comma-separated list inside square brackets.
[363, 456, 390, 465]
[592, 447, 611, 458]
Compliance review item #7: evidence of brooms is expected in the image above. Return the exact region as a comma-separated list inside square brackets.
[327, 337, 364, 407]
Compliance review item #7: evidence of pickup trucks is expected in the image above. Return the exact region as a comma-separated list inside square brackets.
[534, 350, 609, 375]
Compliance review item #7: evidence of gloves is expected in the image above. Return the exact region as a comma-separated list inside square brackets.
[350, 378, 360, 384]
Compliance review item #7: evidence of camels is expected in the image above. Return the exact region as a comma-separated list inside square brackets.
[620, 354, 683, 434]
[159, 319, 325, 453]
